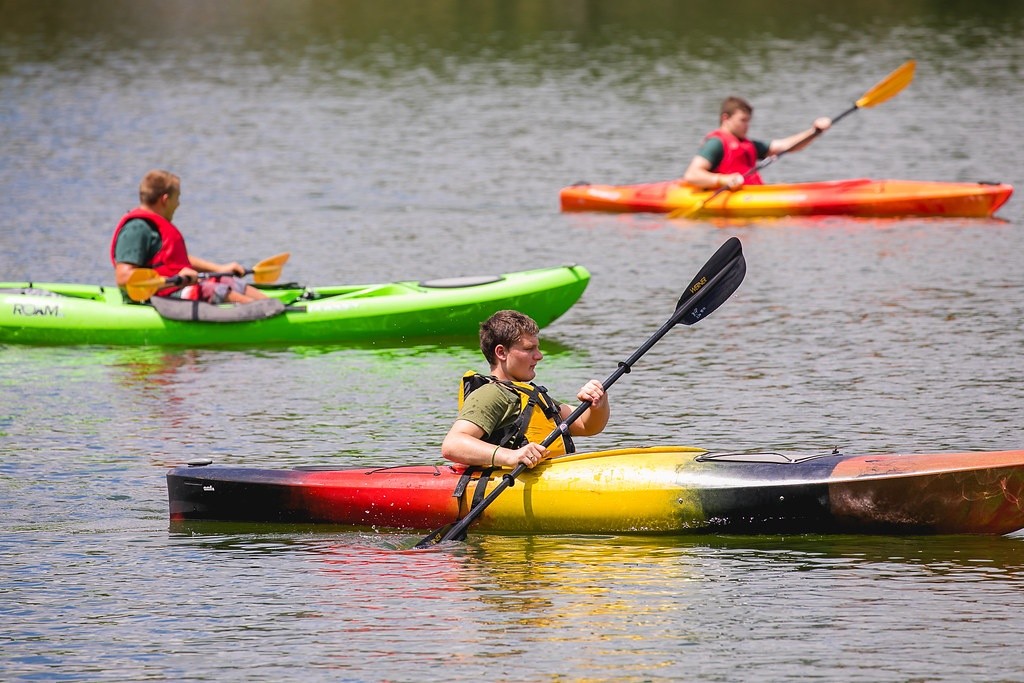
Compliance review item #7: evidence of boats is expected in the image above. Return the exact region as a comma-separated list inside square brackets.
[165, 448, 1024, 534]
[557, 177, 1016, 215]
[1, 262, 593, 347]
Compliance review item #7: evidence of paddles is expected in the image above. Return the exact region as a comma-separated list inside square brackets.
[667, 59, 916, 219]
[413, 233, 750, 551]
[126, 251, 290, 303]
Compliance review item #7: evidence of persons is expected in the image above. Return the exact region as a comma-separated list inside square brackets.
[683, 96, 833, 192]
[110, 168, 270, 305]
[440, 309, 610, 469]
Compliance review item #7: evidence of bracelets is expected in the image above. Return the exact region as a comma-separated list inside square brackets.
[492, 445, 500, 468]
[717, 173, 721, 187]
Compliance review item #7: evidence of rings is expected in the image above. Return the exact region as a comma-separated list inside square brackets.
[531, 457, 532, 459]
